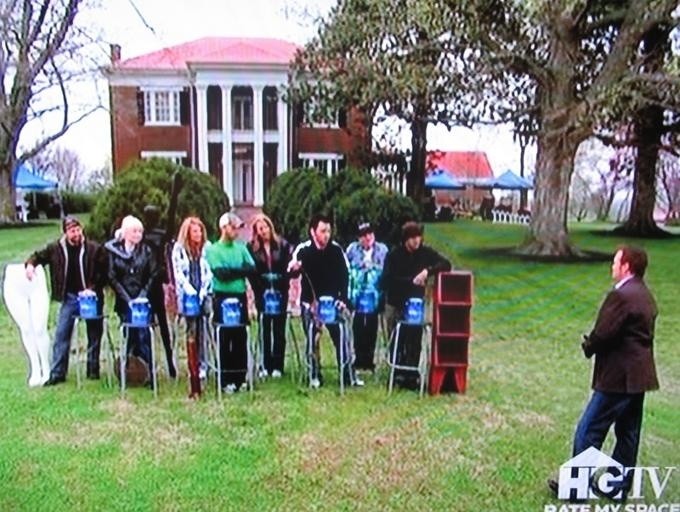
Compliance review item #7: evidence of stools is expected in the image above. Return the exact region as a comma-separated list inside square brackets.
[60, 310, 431, 406]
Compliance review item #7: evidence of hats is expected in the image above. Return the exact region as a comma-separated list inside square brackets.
[353, 218, 374, 238]
[218, 212, 247, 229]
[63, 217, 80, 232]
[121, 214, 143, 232]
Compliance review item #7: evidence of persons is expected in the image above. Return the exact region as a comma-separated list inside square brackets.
[141, 172, 182, 376]
[344, 222, 389, 378]
[105, 216, 157, 366]
[245, 212, 293, 376]
[284, 215, 364, 386]
[172, 218, 214, 377]
[206, 212, 256, 393]
[25, 214, 109, 385]
[547, 245, 660, 501]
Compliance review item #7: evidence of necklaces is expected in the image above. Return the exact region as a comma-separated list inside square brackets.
[383, 220, 452, 386]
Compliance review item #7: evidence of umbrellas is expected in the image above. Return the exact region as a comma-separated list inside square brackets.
[425, 166, 465, 193]
[472, 170, 534, 192]
[15, 164, 57, 188]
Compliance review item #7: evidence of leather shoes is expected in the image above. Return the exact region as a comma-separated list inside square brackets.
[546, 478, 626, 503]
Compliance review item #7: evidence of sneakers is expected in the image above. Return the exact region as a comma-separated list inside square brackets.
[46, 368, 365, 393]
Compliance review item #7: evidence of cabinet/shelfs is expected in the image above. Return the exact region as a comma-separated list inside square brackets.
[427, 268, 473, 397]
